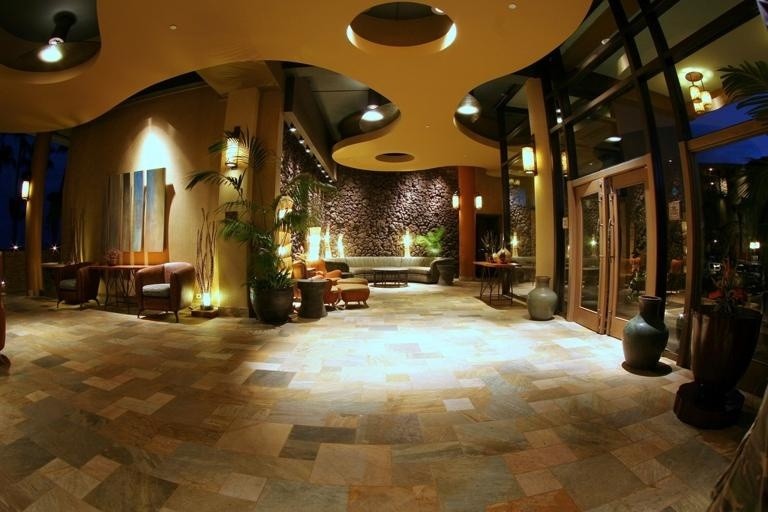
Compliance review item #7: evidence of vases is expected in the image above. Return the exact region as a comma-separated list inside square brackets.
[527, 276, 558, 320]
[623, 295, 669, 371]
[673, 307, 762, 429]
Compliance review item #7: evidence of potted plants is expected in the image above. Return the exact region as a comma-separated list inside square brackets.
[184, 124, 331, 324]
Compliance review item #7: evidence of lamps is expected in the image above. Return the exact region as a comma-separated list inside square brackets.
[21, 179, 29, 200]
[452, 188, 460, 211]
[224, 126, 240, 168]
[521, 143, 538, 175]
[475, 191, 483, 209]
[686, 72, 713, 116]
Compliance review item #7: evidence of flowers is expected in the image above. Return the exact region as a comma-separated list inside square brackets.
[708, 257, 748, 310]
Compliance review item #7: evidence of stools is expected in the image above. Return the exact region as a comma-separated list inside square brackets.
[335, 285, 370, 309]
[336, 277, 368, 286]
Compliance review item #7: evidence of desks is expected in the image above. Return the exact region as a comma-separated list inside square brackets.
[88, 265, 148, 314]
[473, 261, 522, 306]
[297, 279, 329, 318]
[436, 265, 456, 286]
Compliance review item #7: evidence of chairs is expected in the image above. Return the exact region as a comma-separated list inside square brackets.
[55, 261, 101, 311]
[292, 263, 341, 312]
[135, 262, 195, 323]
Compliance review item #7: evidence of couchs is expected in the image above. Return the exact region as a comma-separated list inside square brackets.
[327, 257, 454, 284]
[309, 260, 353, 278]
